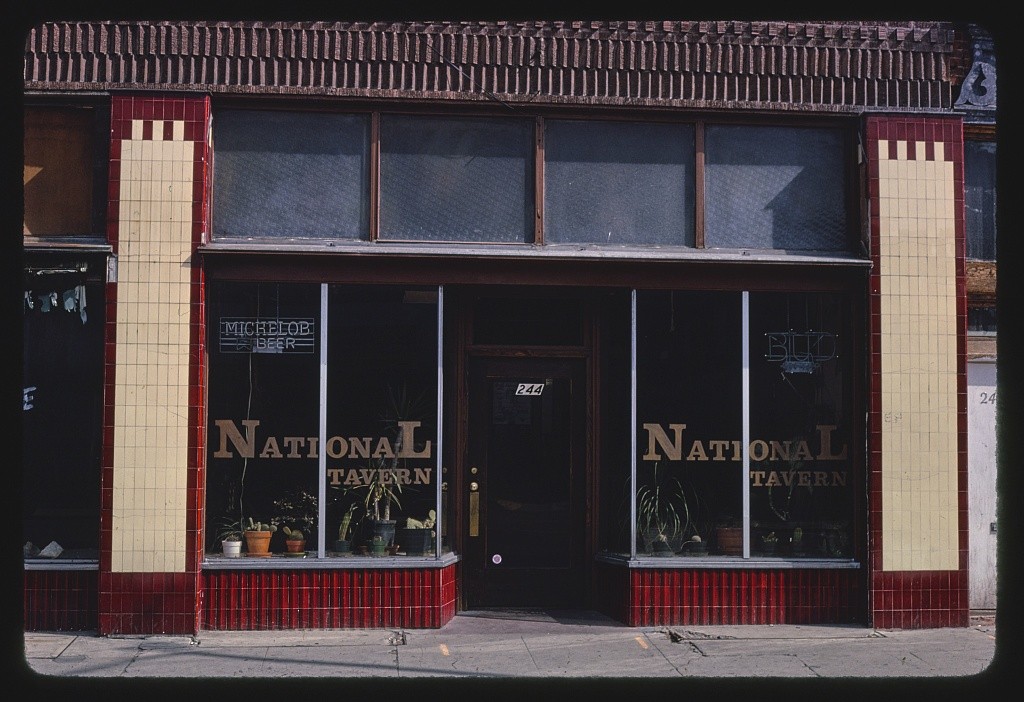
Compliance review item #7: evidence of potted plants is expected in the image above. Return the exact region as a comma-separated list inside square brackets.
[398, 510, 436, 555]
[619, 461, 702, 553]
[218, 522, 243, 559]
[283, 527, 309, 557]
[243, 517, 277, 558]
[368, 536, 385, 556]
[340, 458, 397, 549]
[761, 532, 779, 557]
[782, 528, 816, 557]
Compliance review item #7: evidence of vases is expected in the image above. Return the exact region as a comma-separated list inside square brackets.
[358, 546, 368, 556]
[716, 528, 744, 556]
[818, 532, 852, 558]
[335, 540, 352, 557]
